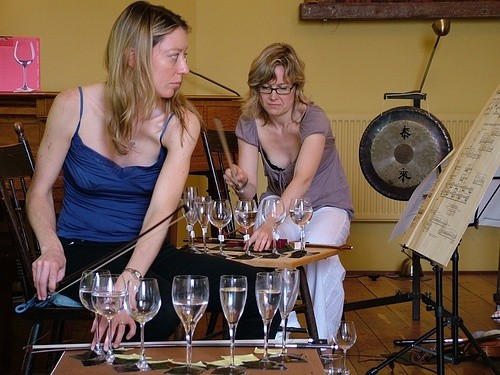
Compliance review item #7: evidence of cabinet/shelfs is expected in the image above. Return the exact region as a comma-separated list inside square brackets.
[0, 92, 249, 368]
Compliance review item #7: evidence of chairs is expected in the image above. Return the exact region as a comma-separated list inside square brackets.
[201, 129, 240, 340]
[0, 121, 94, 375]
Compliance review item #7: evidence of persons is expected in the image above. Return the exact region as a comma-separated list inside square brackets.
[223, 42, 354, 352]
[28, 0, 207, 351]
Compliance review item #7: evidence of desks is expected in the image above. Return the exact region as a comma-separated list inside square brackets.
[177, 243, 352, 339]
[51, 341, 325, 375]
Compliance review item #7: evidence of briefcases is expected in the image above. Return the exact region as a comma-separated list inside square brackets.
[0, 34, 40, 91]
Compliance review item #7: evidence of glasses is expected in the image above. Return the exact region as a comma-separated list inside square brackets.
[258, 82, 296, 95]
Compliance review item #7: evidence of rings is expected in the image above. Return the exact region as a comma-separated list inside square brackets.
[119, 321, 126, 325]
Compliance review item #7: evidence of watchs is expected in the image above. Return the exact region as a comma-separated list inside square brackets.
[123, 268, 143, 283]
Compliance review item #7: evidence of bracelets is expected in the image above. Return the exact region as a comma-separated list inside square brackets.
[232, 180, 250, 193]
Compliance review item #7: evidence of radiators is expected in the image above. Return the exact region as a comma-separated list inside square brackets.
[321, 115, 476, 221]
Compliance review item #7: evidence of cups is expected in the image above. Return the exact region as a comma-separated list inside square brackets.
[321, 353, 344, 375]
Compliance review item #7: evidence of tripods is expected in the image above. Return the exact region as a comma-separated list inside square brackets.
[365, 240, 500, 375]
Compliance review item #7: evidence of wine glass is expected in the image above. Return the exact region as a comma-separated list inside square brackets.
[255, 272, 288, 371]
[183, 185, 199, 242]
[79, 270, 113, 360]
[91, 274, 127, 366]
[208, 200, 233, 259]
[333, 320, 358, 375]
[13, 39, 35, 93]
[182, 196, 202, 254]
[194, 196, 214, 257]
[261, 198, 286, 257]
[234, 199, 259, 258]
[172, 275, 209, 375]
[289, 197, 313, 256]
[217, 274, 248, 375]
[269, 266, 301, 364]
[123, 277, 162, 371]
